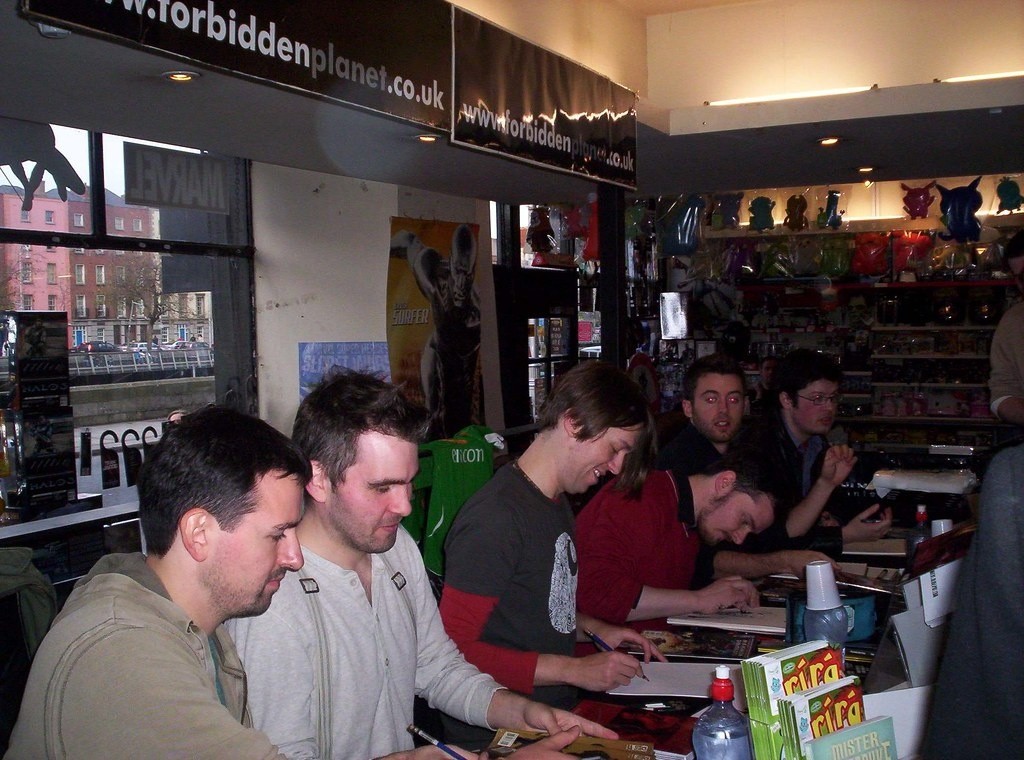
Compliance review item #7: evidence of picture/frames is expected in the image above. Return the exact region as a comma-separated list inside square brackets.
[696, 341, 716, 361]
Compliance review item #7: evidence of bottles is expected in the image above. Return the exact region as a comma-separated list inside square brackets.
[692, 665, 754, 760]
[803, 606, 849, 674]
[910, 504, 931, 558]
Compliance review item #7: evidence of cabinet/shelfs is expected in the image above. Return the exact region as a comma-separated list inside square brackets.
[699, 212, 1024, 456]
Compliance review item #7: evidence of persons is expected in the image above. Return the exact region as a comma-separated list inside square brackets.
[988, 231, 1024, 424]
[439, 361, 667, 738]
[657, 354, 858, 586]
[4, 408, 488, 760]
[224, 373, 619, 760]
[761, 350, 893, 556]
[750, 356, 776, 398]
[576, 451, 789, 656]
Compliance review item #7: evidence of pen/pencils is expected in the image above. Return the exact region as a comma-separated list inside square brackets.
[873, 569, 888, 579]
[582, 627, 650, 682]
[860, 519, 900, 523]
[408, 724, 467, 760]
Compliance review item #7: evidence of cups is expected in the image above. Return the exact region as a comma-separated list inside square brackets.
[932, 519, 953, 538]
[803, 559, 843, 611]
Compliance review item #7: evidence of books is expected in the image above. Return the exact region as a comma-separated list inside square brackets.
[739, 640, 897, 760]
[667, 607, 786, 635]
[842, 540, 906, 556]
[486, 728, 655, 760]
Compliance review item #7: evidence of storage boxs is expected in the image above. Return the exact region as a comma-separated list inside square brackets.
[0, 309, 78, 513]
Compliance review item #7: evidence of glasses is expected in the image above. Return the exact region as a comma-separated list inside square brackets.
[797, 394, 844, 405]
